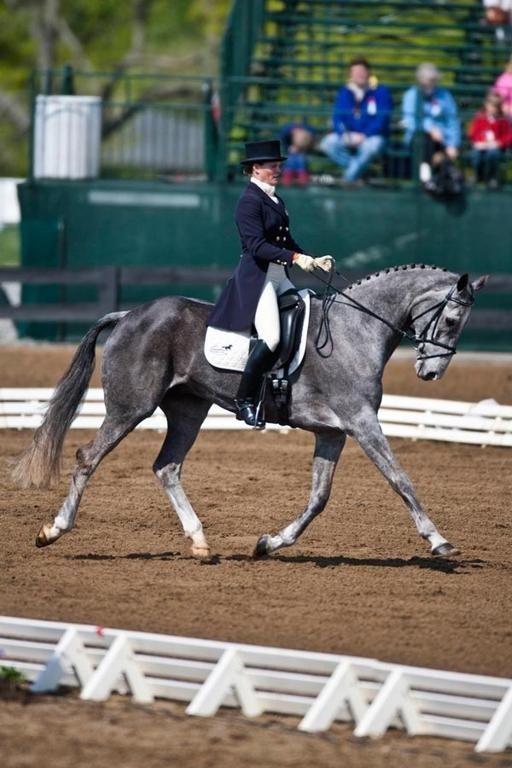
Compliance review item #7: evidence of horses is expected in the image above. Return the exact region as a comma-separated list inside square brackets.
[7, 259, 490, 566]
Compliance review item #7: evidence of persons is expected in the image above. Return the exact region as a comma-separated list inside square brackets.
[465, 59, 512, 194]
[274, 123, 318, 186]
[483, 0, 511, 42]
[398, 61, 462, 200]
[204, 140, 335, 427]
[318, 56, 393, 189]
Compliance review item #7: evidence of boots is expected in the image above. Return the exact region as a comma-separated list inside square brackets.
[237, 339, 273, 425]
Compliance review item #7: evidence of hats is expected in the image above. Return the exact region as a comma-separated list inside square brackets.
[240, 140, 287, 163]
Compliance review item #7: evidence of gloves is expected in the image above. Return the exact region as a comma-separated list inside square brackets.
[315, 255, 335, 271]
[295, 254, 317, 272]
